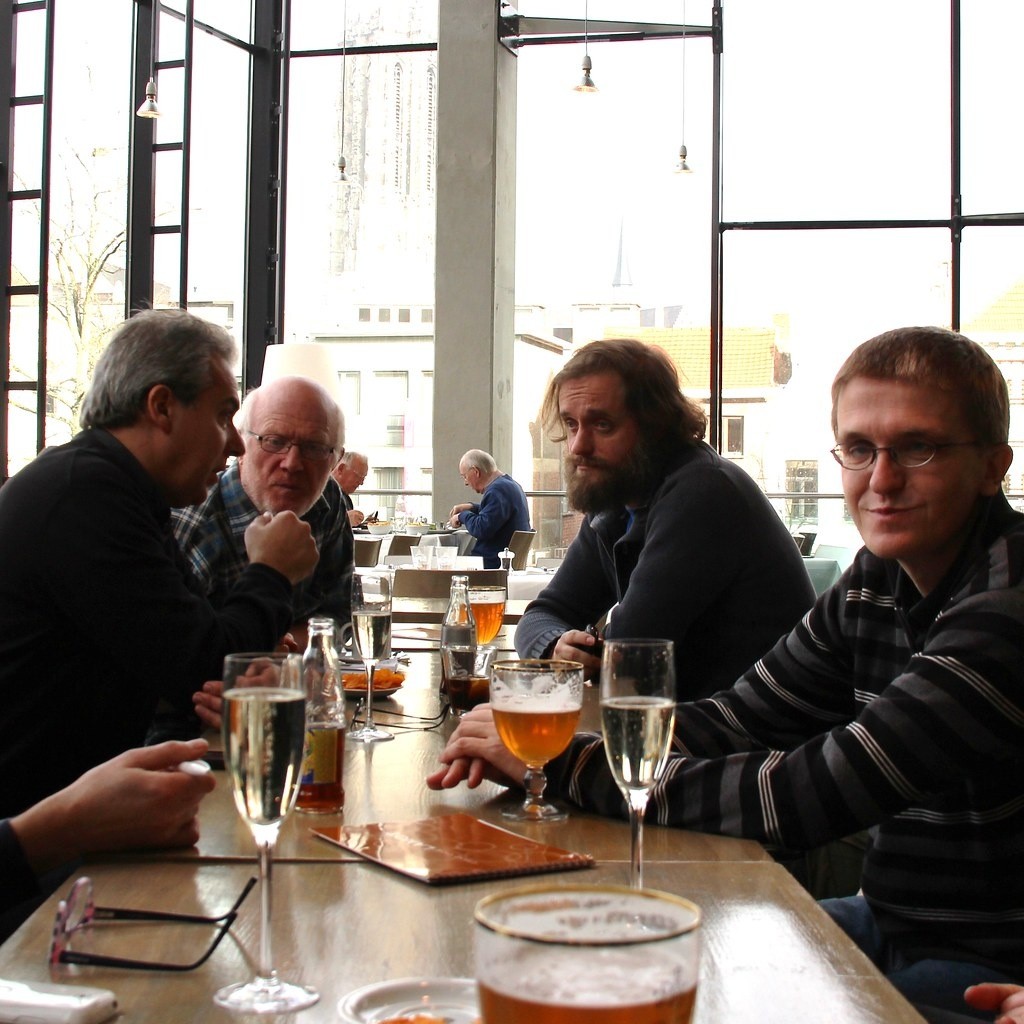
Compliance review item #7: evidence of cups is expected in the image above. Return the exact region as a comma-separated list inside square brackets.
[435, 546, 459, 571]
[410, 546, 434, 570]
[440, 645, 498, 715]
[475, 886, 702, 1024]
[466, 584, 507, 644]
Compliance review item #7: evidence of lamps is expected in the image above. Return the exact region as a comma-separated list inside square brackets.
[334, 0, 352, 182]
[135, 0, 161, 118]
[674, 0, 691, 173]
[575, 1, 600, 93]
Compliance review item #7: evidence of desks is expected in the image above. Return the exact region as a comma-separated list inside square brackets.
[353, 528, 466, 558]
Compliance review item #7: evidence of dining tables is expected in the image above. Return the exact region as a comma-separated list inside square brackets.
[0, 611, 932, 1024]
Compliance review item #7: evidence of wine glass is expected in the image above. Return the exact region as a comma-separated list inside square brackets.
[212, 653, 323, 1016]
[489, 658, 584, 822]
[599, 638, 675, 887]
[345, 572, 394, 741]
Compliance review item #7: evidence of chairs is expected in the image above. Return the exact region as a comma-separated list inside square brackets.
[352, 526, 538, 599]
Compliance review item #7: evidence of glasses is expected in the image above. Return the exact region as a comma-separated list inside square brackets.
[350, 697, 452, 732]
[352, 469, 368, 480]
[828, 439, 984, 471]
[49, 875, 258, 973]
[247, 430, 337, 463]
[460, 468, 472, 478]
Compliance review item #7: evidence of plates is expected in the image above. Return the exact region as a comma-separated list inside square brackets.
[336, 975, 479, 1024]
[352, 530, 369, 534]
[429, 529, 457, 534]
[344, 684, 404, 699]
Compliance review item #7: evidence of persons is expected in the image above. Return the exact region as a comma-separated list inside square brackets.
[170, 376, 369, 654]
[448, 449, 531, 569]
[965, 983, 1024, 1024]
[424, 326, 1024, 1024]
[0, 308, 321, 817]
[0, 738, 218, 946]
[515, 340, 875, 901]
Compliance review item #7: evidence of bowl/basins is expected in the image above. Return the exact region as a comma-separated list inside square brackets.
[406, 525, 429, 535]
[367, 524, 392, 534]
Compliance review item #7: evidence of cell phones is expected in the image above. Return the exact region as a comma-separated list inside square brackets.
[0, 978, 118, 1024]
[201, 751, 225, 771]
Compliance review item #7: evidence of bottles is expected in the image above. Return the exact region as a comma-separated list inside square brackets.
[293, 618, 345, 813]
[395, 495, 406, 527]
[439, 576, 478, 701]
